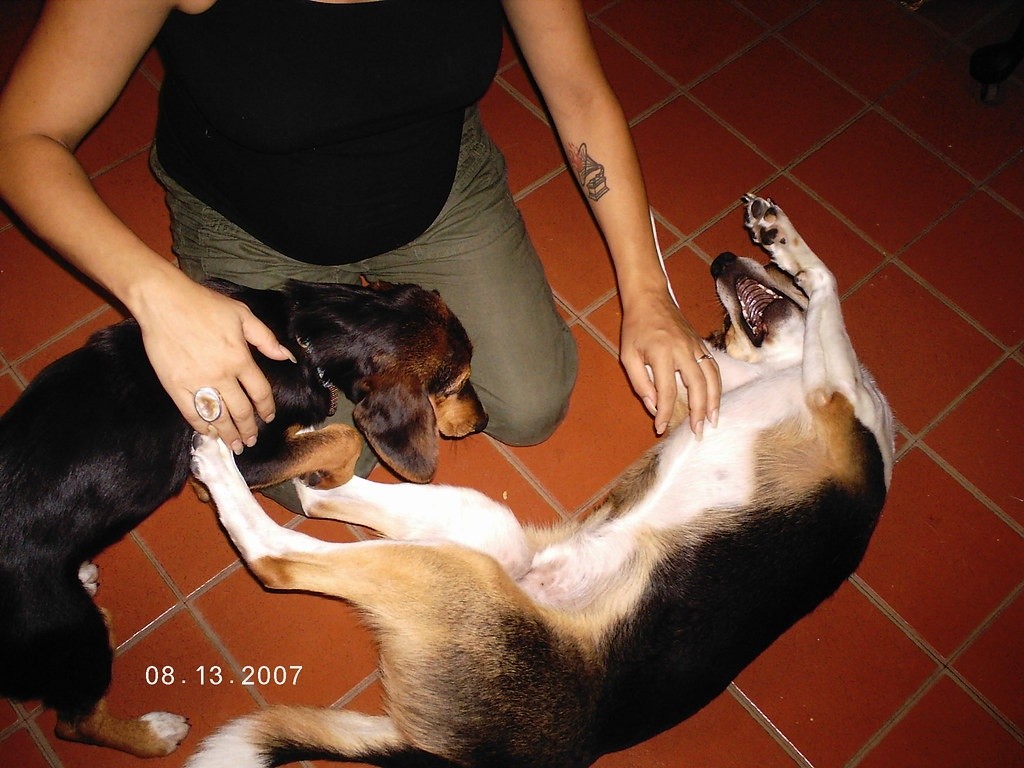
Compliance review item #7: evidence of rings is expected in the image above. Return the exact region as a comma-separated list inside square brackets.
[195, 387, 222, 422]
[697, 354, 713, 364]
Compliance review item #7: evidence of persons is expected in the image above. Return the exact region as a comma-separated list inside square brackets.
[0, 0, 722, 517]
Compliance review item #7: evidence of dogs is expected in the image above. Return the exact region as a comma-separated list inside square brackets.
[0, 272, 490, 760]
[180, 192, 897, 768]
